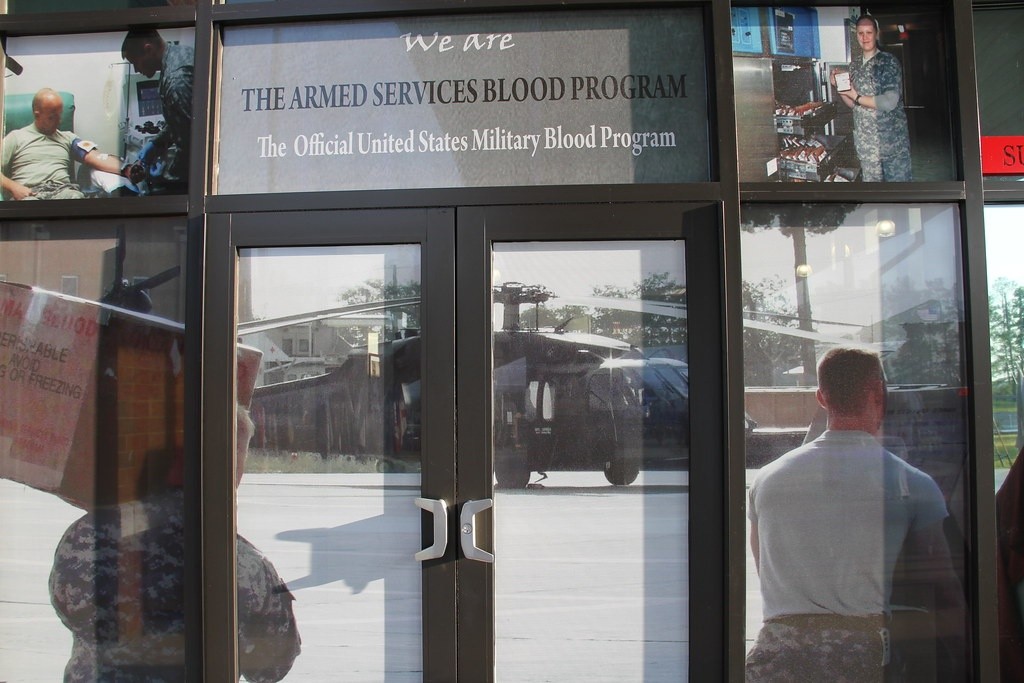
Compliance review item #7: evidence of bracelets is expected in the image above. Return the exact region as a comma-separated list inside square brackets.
[120, 163, 132, 176]
[855, 94, 861, 106]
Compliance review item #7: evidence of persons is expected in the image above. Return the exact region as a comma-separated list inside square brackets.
[745, 345, 973, 683]
[49, 368, 301, 683]
[828, 15, 912, 183]
[120, 23, 195, 177]
[0, 91, 144, 201]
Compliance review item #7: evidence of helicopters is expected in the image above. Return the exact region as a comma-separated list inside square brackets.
[230, 278, 882, 491]
